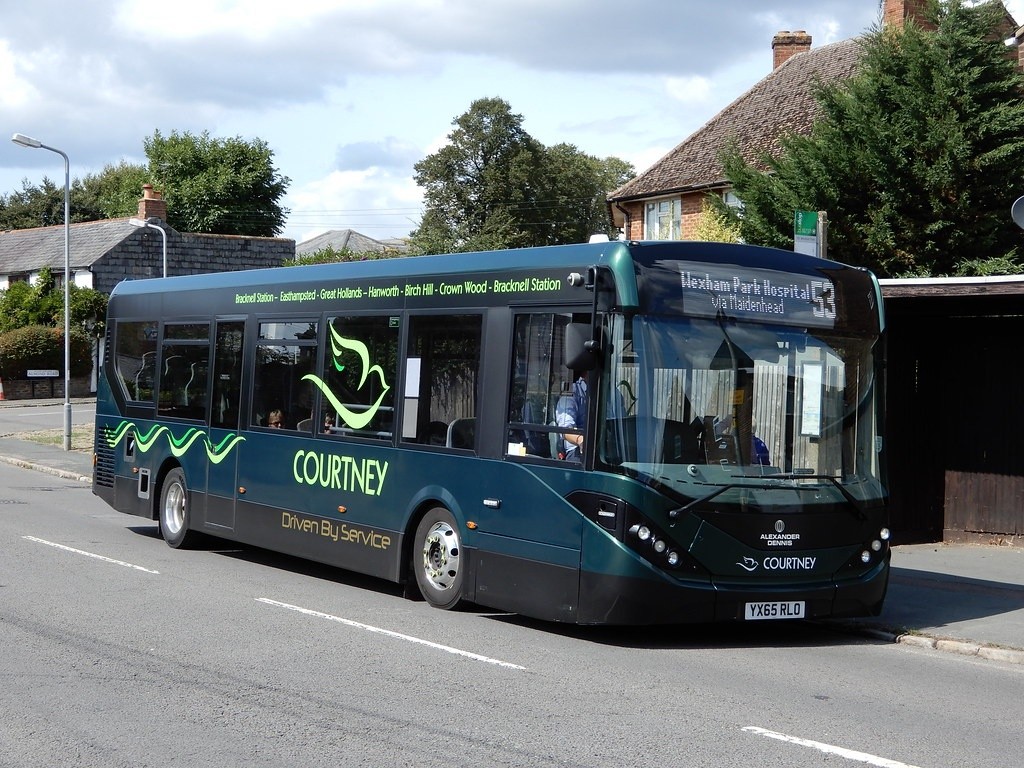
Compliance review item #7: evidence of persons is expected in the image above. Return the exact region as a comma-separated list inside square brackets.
[268, 408, 288, 430]
[556, 365, 627, 464]
[324, 412, 337, 434]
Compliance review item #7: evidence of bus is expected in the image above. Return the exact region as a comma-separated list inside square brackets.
[89, 240, 895, 639]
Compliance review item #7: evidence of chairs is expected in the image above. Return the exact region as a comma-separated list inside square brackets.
[161, 356, 188, 406]
[417, 415, 477, 449]
[545, 395, 564, 461]
[184, 361, 209, 412]
[222, 383, 238, 426]
[135, 351, 165, 401]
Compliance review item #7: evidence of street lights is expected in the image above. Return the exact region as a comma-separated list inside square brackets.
[11, 133, 71, 452]
